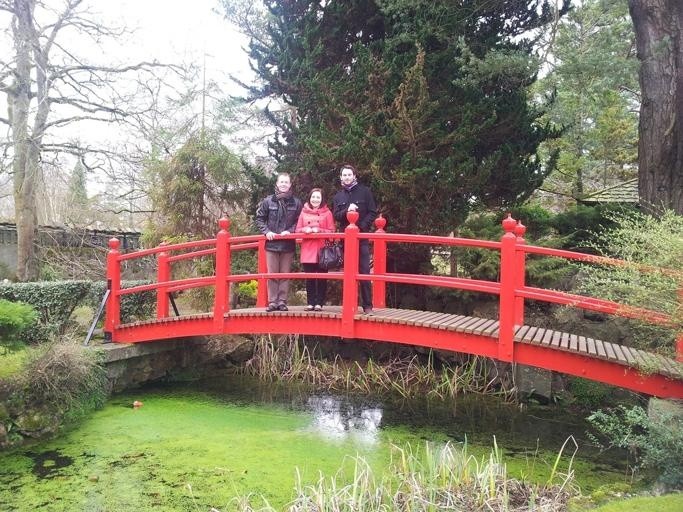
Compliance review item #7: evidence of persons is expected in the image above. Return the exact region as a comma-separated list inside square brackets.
[333, 164, 380, 316]
[255, 173, 305, 312]
[294, 187, 338, 312]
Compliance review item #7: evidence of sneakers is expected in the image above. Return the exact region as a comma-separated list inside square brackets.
[266, 305, 276, 312]
[277, 304, 288, 311]
[304, 305, 314, 311]
[363, 307, 374, 316]
[314, 304, 322, 311]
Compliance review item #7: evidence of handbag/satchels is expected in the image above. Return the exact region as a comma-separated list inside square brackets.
[317, 238, 344, 271]
